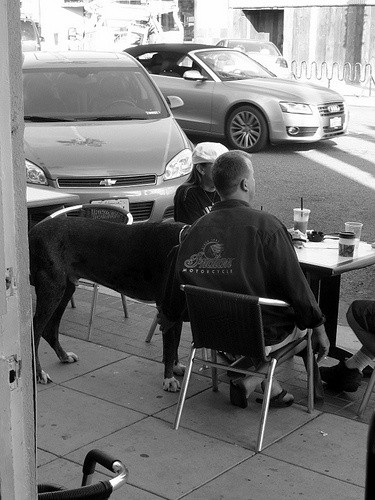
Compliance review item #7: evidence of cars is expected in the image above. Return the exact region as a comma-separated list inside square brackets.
[21, 47, 196, 224]
[121, 44, 348, 154]
[217, 38, 286, 67]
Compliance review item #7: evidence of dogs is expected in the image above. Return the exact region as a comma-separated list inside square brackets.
[29, 217, 194, 392]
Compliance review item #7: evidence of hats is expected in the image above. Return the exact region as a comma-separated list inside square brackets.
[192, 142, 230, 165]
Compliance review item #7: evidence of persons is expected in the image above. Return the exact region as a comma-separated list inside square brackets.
[318, 242, 375, 393]
[174, 150, 331, 409]
[173, 142, 230, 225]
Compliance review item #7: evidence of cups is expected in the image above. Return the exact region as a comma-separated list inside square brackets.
[338, 231, 356, 263]
[293, 207, 311, 235]
[345, 221, 364, 255]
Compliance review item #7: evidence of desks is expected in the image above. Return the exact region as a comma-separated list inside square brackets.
[26, 186, 78, 207]
[294, 233, 375, 377]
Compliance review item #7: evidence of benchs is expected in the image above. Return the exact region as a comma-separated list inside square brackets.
[59, 83, 97, 114]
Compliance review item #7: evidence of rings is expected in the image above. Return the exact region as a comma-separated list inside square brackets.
[323, 356, 327, 360]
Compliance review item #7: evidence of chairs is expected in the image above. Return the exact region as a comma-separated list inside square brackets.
[144, 217, 207, 368]
[23, 72, 67, 114]
[148, 54, 166, 74]
[33, 204, 134, 340]
[93, 78, 133, 113]
[174, 284, 315, 452]
[38, 449, 127, 500]
[191, 58, 205, 73]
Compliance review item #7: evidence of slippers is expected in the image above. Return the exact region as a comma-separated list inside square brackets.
[229, 379, 248, 408]
[218, 351, 245, 363]
[255, 388, 295, 408]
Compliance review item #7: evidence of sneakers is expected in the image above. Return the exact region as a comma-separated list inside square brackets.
[318, 356, 360, 392]
[360, 364, 375, 379]
